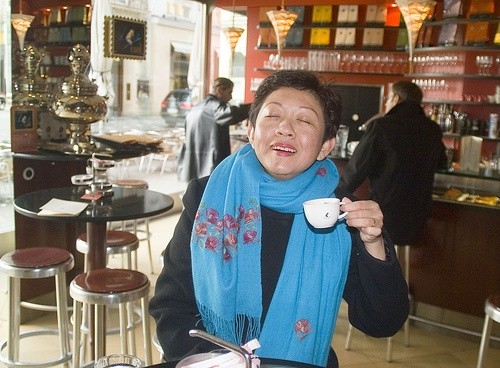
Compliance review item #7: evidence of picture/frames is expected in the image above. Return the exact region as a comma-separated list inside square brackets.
[103, 15, 147, 60]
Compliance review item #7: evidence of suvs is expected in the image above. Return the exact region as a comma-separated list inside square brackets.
[160, 90, 192, 128]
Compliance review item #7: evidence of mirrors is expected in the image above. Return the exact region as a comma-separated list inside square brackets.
[9, 0, 92, 110]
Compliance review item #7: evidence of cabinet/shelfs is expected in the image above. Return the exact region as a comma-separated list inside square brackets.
[30, 22, 91, 67]
[254, 17, 500, 141]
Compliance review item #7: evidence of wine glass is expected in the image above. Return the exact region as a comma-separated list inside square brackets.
[340, 54, 408, 73]
[409, 55, 458, 100]
[476, 56, 500, 75]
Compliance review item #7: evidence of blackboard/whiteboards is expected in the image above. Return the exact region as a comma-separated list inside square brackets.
[328, 83, 385, 143]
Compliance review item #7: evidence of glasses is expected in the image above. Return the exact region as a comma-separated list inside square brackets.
[383, 94, 401, 101]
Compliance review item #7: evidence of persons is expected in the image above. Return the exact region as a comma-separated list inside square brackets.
[338, 79, 443, 243]
[147, 69, 411, 368]
[176, 77, 253, 183]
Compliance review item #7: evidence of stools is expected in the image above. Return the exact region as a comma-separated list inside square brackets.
[0, 180, 154, 368]
[477, 293, 500, 368]
[344, 239, 411, 362]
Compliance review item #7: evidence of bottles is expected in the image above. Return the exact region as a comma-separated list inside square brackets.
[335, 125, 348, 158]
[428, 104, 465, 134]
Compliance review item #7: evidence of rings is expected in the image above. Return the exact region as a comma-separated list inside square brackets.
[371, 218, 377, 227]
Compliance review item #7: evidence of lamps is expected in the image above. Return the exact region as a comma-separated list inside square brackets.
[222, 0, 245, 68]
[266, 0, 299, 60]
[394, 0, 437, 75]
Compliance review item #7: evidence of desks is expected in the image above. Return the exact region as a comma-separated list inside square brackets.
[13, 185, 173, 361]
[10, 146, 174, 161]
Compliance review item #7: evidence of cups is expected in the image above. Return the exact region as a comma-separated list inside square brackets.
[347, 141, 360, 152]
[465, 113, 500, 139]
[303, 198, 349, 228]
[95, 354, 145, 368]
[465, 85, 500, 103]
[265, 51, 340, 72]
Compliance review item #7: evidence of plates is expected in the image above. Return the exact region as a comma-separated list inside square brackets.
[347, 152, 353, 155]
